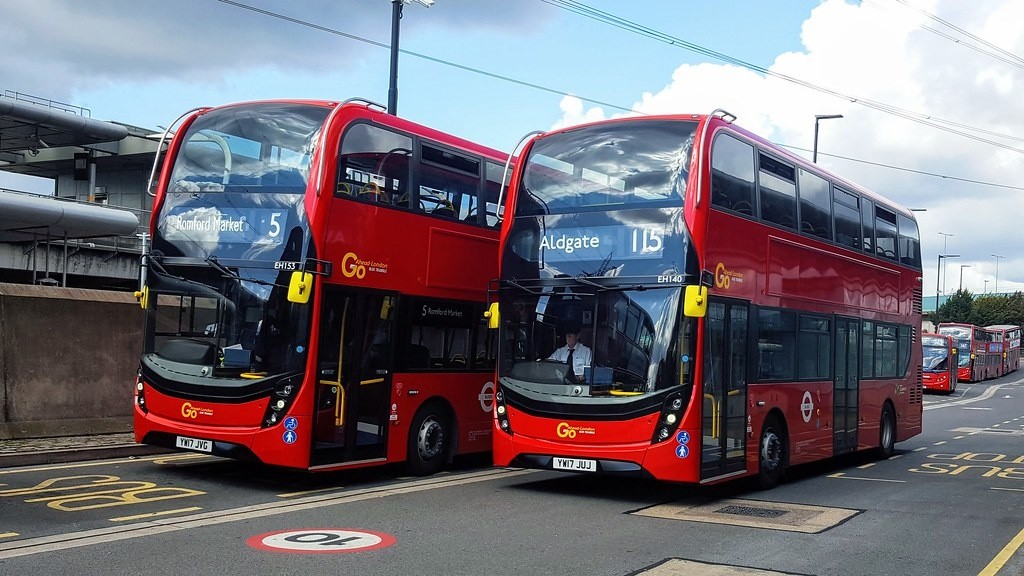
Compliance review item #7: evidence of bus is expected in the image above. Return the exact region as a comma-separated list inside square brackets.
[131, 98, 516, 476]
[937, 322, 1020, 383]
[922, 333, 959, 392]
[482, 115, 924, 487]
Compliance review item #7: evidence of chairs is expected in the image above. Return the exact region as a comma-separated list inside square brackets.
[230, 166, 903, 399]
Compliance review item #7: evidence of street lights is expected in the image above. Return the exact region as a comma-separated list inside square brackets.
[812, 113, 844, 165]
[960, 264, 970, 291]
[991, 253, 1005, 293]
[984, 280, 989, 294]
[936, 253, 960, 313]
[939, 231, 953, 303]
[908, 208, 927, 212]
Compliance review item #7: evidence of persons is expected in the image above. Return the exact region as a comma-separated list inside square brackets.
[546, 325, 593, 381]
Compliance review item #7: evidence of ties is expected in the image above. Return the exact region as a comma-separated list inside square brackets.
[565, 349, 575, 380]
